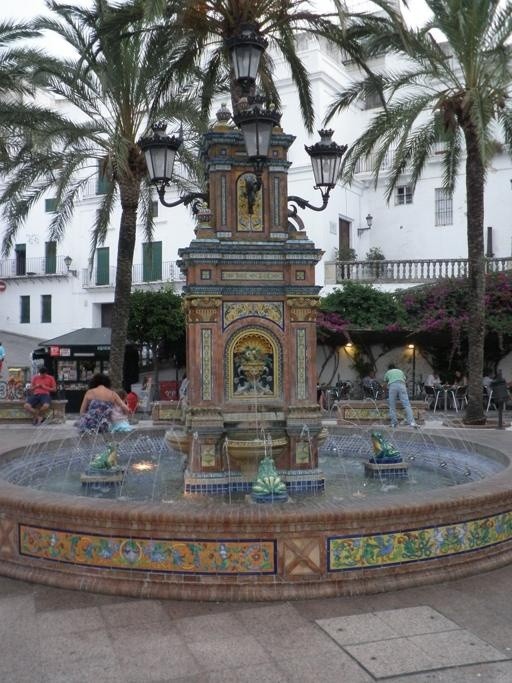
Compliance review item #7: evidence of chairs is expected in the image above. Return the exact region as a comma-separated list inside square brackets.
[317, 375, 512, 416]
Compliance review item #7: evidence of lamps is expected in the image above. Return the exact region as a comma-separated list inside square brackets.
[357, 214, 375, 236]
[64, 255, 77, 279]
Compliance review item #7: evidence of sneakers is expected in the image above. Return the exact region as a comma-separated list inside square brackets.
[389, 423, 398, 427]
[32, 416, 43, 426]
[411, 422, 420, 428]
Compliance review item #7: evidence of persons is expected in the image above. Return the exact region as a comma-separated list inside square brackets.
[384, 364, 421, 430]
[362, 370, 381, 398]
[24, 368, 57, 425]
[73, 373, 133, 435]
[0, 343, 6, 377]
[179, 372, 187, 424]
[123, 385, 139, 412]
[453, 370, 467, 387]
[424, 369, 439, 411]
[483, 368, 494, 404]
